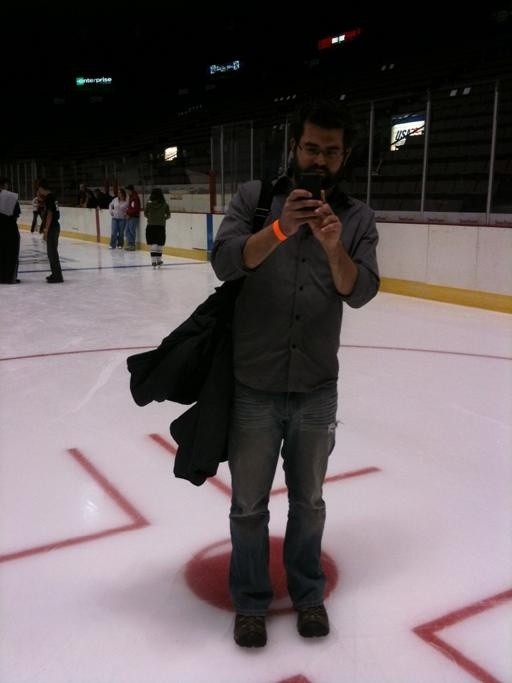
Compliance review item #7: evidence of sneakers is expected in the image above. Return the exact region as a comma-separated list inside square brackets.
[296, 604, 331, 636]
[233, 614, 268, 648]
[108, 245, 137, 251]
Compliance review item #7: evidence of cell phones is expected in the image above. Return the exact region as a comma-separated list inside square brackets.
[299, 173, 321, 202]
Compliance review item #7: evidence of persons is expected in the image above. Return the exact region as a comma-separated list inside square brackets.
[206, 93, 382, 646]
[1, 176, 171, 284]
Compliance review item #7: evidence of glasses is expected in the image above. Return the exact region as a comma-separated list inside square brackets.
[291, 136, 347, 161]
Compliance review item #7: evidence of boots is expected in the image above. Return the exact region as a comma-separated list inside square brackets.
[151, 255, 163, 266]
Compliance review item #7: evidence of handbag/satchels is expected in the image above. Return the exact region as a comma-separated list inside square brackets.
[164, 297, 226, 405]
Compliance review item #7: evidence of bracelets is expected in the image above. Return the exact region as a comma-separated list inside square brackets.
[272, 219, 288, 242]
